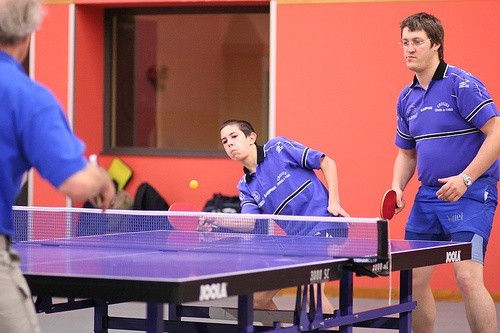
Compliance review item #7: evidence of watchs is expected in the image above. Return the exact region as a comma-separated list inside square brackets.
[460, 173, 472, 187]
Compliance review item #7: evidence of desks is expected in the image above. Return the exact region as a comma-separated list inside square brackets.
[10, 206, 472, 333]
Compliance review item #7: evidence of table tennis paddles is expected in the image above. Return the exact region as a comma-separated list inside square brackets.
[378, 189, 404, 220]
[87, 153, 104, 209]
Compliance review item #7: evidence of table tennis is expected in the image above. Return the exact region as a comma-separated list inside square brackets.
[188, 180, 198, 188]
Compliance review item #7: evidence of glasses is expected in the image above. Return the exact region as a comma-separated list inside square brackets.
[398, 36, 432, 47]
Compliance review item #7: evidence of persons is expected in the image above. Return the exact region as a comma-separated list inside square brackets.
[199, 120, 352, 330]
[0, 0, 113, 333]
[391, 12, 500, 333]
